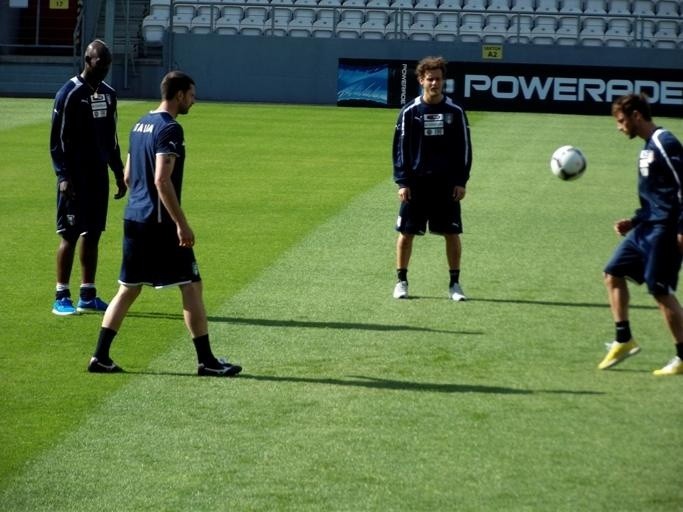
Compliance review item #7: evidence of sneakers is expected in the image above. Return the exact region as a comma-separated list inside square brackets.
[76, 298, 107, 313]
[393, 281, 408, 299]
[598, 338, 640, 368]
[449, 283, 466, 301]
[653, 356, 683, 375]
[198, 358, 242, 377]
[88, 356, 122, 372]
[52, 297, 79, 315]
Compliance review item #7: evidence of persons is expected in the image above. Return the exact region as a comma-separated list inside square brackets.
[50, 38, 124, 318]
[599, 93, 683, 377]
[89, 70, 242, 377]
[392, 53, 474, 301]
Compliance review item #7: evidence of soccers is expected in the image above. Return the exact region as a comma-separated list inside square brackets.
[551, 145, 586, 181]
